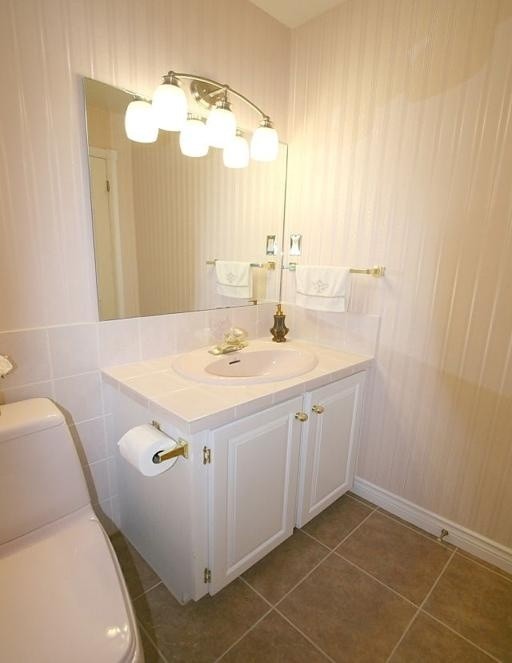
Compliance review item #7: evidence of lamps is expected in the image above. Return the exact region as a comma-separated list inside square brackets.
[154, 71, 279, 165]
[124, 95, 249, 170]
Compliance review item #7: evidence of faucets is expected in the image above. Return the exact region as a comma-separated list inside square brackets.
[209, 329, 247, 356]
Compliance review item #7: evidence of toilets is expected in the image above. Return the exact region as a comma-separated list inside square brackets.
[0, 398, 145, 663]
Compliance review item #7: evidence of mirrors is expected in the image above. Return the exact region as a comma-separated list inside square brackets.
[82, 75, 289, 323]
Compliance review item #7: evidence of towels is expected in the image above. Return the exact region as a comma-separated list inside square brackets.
[215, 260, 253, 299]
[295, 265, 349, 312]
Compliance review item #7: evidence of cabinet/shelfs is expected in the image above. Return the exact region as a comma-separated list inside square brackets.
[103, 371, 365, 608]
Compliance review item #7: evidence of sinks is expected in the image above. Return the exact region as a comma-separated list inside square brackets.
[174, 342, 318, 383]
